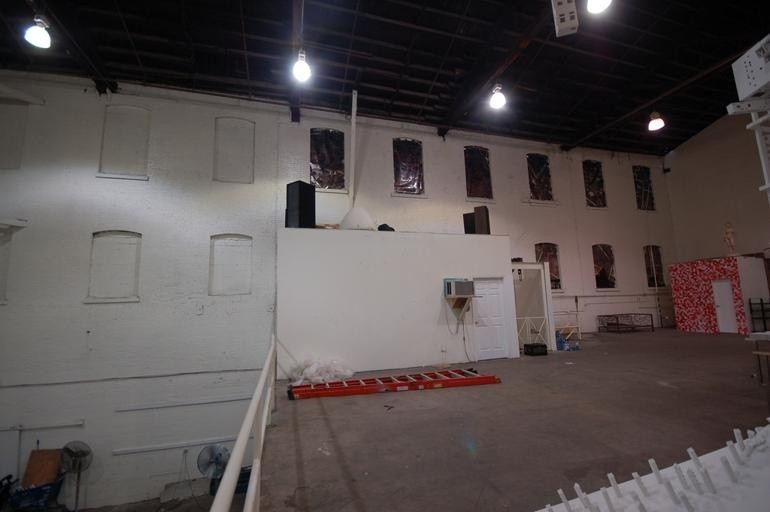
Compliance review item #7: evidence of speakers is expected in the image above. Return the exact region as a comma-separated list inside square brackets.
[286, 180, 316, 228]
[474, 205, 491, 234]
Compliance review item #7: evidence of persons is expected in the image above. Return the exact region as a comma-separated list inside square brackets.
[723, 222, 736, 247]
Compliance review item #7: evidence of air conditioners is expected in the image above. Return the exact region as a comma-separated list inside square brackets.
[444, 277, 474, 299]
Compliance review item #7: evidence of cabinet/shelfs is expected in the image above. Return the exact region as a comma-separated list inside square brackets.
[748, 296, 770, 332]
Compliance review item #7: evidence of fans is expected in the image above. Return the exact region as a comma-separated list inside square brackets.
[198, 442, 237, 494]
[60, 440, 92, 511]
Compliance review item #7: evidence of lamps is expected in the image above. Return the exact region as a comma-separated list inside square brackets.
[292, 48, 312, 83]
[488, 83, 507, 110]
[646, 105, 666, 132]
[23, 13, 52, 49]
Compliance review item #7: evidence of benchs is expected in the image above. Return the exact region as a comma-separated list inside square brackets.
[598, 312, 654, 334]
